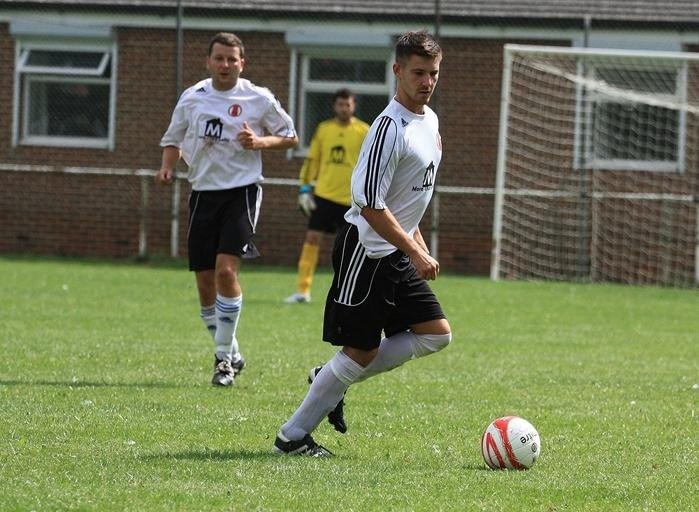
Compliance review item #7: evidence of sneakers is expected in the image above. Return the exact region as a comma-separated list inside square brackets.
[307, 365, 348, 434]
[212, 353, 234, 387]
[285, 292, 312, 304]
[271, 431, 337, 459]
[230, 356, 246, 379]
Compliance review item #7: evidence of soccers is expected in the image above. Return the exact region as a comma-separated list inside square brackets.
[481, 417, 542, 470]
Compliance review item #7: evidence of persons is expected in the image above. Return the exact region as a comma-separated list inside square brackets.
[273, 31, 452, 459]
[284, 89, 370, 304]
[154, 32, 299, 386]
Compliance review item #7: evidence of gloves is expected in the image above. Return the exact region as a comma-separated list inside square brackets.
[297, 186, 317, 218]
